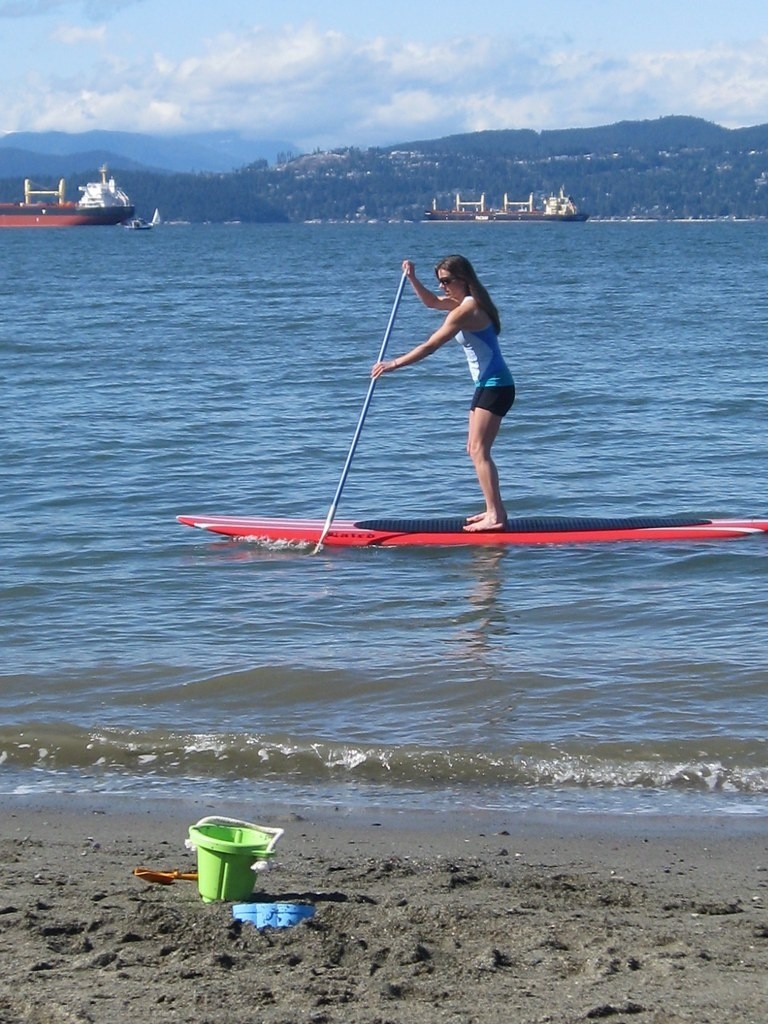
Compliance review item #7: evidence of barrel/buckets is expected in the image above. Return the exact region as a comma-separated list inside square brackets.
[184, 816, 285, 902]
[232, 903, 316, 929]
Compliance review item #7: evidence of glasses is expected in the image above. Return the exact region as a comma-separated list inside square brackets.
[439, 277, 461, 285]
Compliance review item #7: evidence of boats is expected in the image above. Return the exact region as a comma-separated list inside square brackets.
[0, 164, 135, 227]
[124, 219, 153, 230]
[424, 187, 590, 222]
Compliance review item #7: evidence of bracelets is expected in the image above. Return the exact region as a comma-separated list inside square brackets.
[393, 360, 399, 368]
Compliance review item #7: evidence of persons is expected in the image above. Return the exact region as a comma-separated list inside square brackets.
[369, 255, 516, 532]
